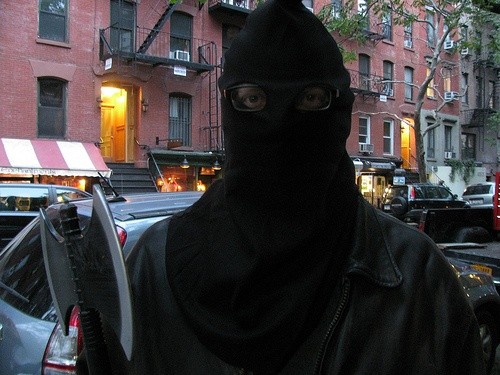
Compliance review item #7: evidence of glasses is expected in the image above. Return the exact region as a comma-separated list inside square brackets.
[224, 85, 336, 113]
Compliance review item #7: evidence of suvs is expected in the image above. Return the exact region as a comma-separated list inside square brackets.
[382, 182, 500, 237]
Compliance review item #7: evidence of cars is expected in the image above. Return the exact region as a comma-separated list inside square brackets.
[0, 183, 210, 375]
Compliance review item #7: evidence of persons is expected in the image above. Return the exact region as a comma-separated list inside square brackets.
[76, 0, 486, 375]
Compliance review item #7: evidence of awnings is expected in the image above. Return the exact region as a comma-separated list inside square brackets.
[0, 137, 111, 179]
